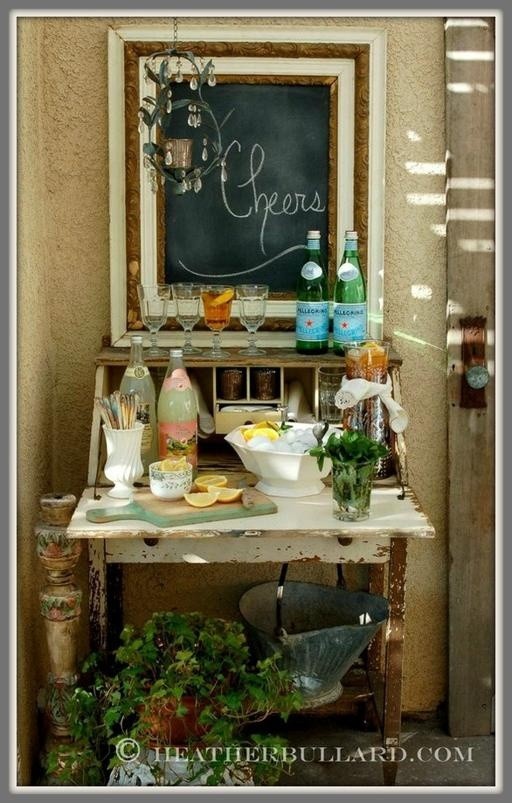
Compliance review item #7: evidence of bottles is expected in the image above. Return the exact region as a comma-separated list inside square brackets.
[119, 332, 201, 481]
[295, 227, 370, 353]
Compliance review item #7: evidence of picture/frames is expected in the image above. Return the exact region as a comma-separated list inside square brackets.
[104, 21, 390, 350]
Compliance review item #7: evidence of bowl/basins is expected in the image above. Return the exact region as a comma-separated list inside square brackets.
[222, 420, 345, 498]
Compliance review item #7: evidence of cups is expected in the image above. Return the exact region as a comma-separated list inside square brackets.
[146, 458, 193, 503]
[340, 335, 393, 480]
[253, 367, 278, 400]
[222, 367, 242, 400]
[318, 367, 354, 421]
[327, 456, 374, 523]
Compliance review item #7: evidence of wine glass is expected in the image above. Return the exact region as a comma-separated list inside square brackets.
[102, 419, 147, 499]
[136, 282, 269, 356]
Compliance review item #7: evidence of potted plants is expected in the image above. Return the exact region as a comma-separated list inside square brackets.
[300, 418, 392, 522]
[42, 606, 312, 785]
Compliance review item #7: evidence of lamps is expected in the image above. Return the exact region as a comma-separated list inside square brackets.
[136, 16, 228, 196]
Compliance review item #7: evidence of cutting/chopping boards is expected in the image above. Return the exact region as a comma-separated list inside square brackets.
[84, 478, 279, 528]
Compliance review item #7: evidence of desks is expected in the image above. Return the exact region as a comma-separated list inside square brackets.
[59, 340, 438, 786]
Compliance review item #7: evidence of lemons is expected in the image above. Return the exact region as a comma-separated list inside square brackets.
[207, 485, 245, 504]
[183, 491, 220, 509]
[239, 420, 280, 443]
[194, 474, 228, 493]
[159, 457, 187, 473]
[211, 288, 235, 307]
